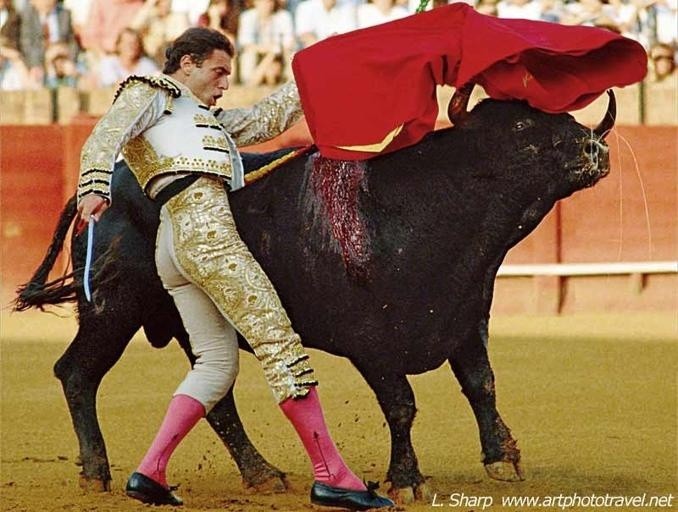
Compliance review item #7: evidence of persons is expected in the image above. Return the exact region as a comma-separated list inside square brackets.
[74, 25, 395, 512]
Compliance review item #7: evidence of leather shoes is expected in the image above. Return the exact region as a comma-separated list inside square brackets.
[311, 482, 394, 509]
[127, 472, 183, 505]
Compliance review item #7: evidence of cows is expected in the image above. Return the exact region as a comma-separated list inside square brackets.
[9, 83, 618, 508]
